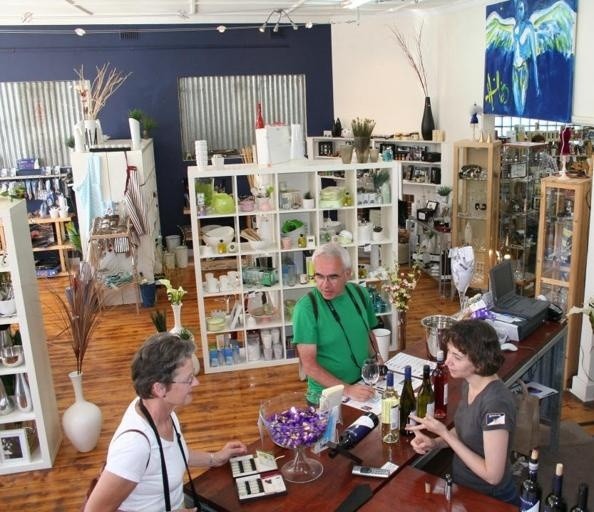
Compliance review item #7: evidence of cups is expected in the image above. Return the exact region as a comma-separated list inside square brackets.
[50, 206, 68, 218]
[240, 199, 254, 212]
[370, 149, 378, 162]
[208, 327, 283, 368]
[283, 297, 295, 320]
[195, 138, 208, 172]
[281, 237, 292, 250]
[431, 129, 443, 142]
[204, 270, 239, 294]
[164, 234, 187, 271]
[371, 327, 391, 362]
[340, 144, 353, 163]
[389, 127, 421, 142]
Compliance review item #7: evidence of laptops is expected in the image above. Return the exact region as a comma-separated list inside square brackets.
[490, 261, 551, 320]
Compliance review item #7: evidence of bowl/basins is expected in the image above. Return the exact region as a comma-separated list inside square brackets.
[202, 234, 234, 247]
[207, 317, 226, 331]
[420, 314, 460, 360]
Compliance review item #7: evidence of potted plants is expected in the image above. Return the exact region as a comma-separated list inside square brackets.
[142, 119, 161, 140]
[127, 107, 145, 140]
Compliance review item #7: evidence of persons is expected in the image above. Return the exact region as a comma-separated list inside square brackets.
[405, 319, 521, 511]
[527, 135, 558, 177]
[81, 332, 246, 512]
[291, 242, 387, 403]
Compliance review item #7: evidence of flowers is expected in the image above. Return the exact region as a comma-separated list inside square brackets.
[160, 277, 188, 305]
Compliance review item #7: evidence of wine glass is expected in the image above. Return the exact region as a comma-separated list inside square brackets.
[362, 357, 379, 404]
[256, 391, 330, 483]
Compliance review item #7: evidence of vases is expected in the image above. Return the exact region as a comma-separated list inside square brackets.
[420, 96, 435, 140]
[83, 119, 99, 144]
[167, 305, 196, 343]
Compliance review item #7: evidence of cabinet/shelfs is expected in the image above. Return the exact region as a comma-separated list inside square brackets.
[304, 138, 453, 277]
[536, 171, 591, 394]
[68, 140, 164, 307]
[453, 139, 534, 301]
[1, 193, 62, 476]
[188, 162, 403, 372]
[2, 165, 77, 279]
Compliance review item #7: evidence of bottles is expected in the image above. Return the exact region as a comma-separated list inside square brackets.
[357, 264, 388, 280]
[210, 153, 225, 169]
[431, 167, 441, 183]
[218, 239, 226, 254]
[255, 103, 263, 129]
[278, 181, 302, 210]
[461, 292, 488, 320]
[325, 412, 378, 459]
[380, 350, 449, 445]
[518, 447, 591, 512]
[355, 187, 384, 205]
[296, 232, 305, 249]
[332, 118, 343, 138]
[342, 191, 353, 208]
[285, 253, 316, 287]
[382, 146, 391, 161]
[0, 325, 31, 416]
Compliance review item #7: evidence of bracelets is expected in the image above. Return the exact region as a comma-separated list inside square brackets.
[208, 452, 215, 468]
[376, 352, 380, 356]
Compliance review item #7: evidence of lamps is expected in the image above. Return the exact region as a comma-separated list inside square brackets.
[257, 9, 300, 33]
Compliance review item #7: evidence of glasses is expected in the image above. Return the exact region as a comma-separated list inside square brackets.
[313, 271, 345, 282]
[158, 368, 195, 386]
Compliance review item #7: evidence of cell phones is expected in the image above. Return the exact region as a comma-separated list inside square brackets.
[352, 465, 390, 478]
